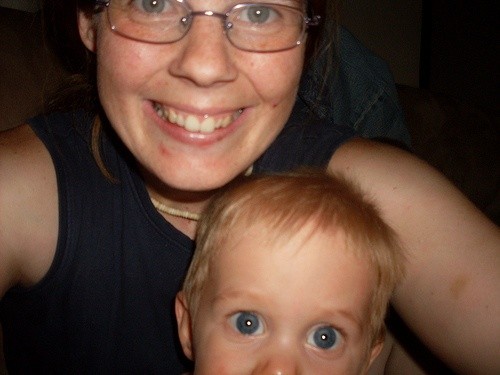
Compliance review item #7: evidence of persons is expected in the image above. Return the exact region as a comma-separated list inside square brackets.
[0, 0, 500, 375]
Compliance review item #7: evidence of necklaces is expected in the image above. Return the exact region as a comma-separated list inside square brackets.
[150, 197, 213, 221]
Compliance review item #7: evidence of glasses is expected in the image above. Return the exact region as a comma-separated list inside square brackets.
[96, 0, 321, 53]
